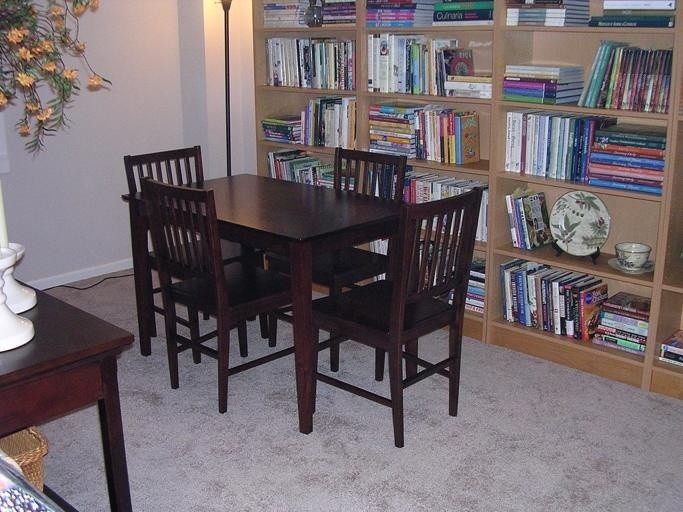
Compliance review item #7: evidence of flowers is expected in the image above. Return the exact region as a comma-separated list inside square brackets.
[0, 0, 112, 157]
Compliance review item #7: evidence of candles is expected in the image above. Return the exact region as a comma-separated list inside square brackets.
[0, 181, 9, 248]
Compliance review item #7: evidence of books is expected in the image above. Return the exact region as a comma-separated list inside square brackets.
[505, 108, 668, 196]
[506, 0, 676, 27]
[504, 40, 672, 113]
[262, 0, 357, 190]
[506, 193, 554, 250]
[500, 258, 652, 358]
[659, 329, 683, 366]
[370, 238, 486, 313]
[365, 0, 494, 240]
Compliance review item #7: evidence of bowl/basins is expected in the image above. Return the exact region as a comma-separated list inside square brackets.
[616, 242, 651, 269]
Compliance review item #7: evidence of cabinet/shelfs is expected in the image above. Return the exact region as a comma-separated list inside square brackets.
[492, 0, 682, 390]
[251, 0, 361, 292]
[355, 0, 498, 348]
[644, 1, 683, 402]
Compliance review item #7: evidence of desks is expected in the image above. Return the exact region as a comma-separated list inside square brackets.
[121, 173, 418, 435]
[1, 273, 134, 510]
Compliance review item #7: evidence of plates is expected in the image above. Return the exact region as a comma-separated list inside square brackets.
[607, 257, 656, 274]
[549, 191, 610, 257]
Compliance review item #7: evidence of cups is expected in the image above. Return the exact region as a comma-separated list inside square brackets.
[303, 6, 322, 28]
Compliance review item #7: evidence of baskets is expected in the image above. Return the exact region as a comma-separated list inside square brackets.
[0, 426, 49, 493]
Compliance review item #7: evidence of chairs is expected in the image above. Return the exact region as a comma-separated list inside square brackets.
[265, 146, 408, 372]
[140, 175, 292, 414]
[124, 144, 243, 338]
[312, 186, 483, 449]
